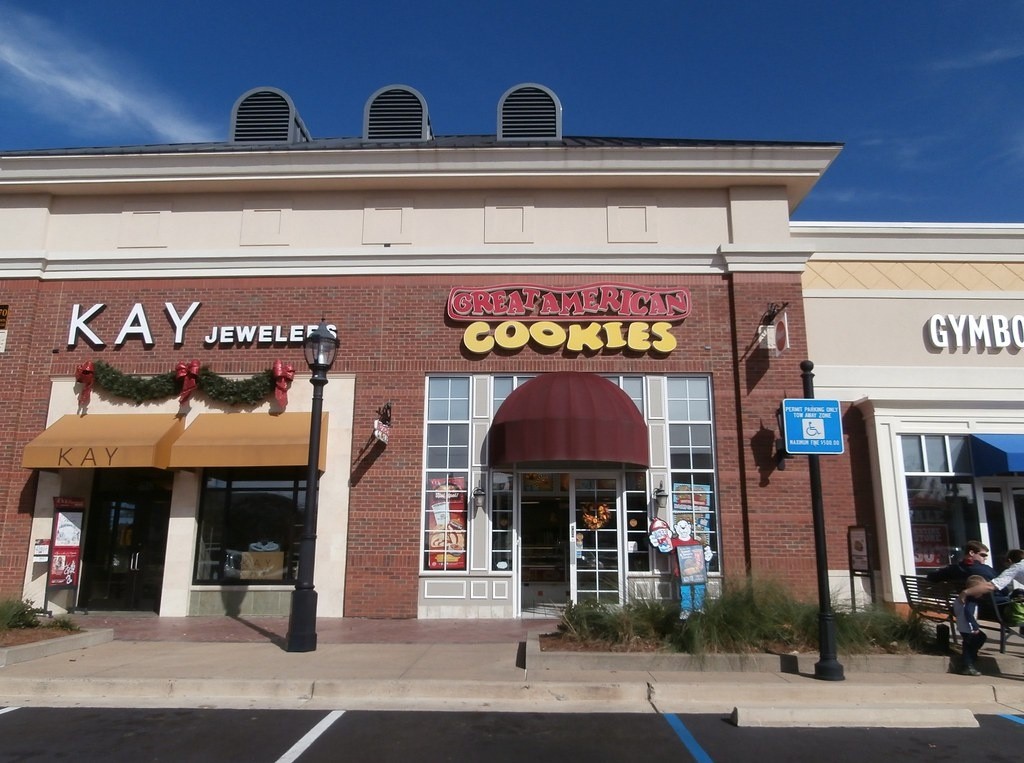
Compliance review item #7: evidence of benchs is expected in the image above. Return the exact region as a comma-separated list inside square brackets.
[901, 574, 1024, 654]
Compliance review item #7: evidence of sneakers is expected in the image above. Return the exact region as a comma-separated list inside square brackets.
[960, 662, 981, 676]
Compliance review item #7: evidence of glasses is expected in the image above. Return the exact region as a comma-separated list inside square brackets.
[977, 553, 988, 557]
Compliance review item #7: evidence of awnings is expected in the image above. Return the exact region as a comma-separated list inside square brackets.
[21, 412, 329, 472]
[970, 433, 1023, 473]
[488, 372, 650, 468]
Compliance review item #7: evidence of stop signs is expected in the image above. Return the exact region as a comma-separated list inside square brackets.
[775, 321, 789, 353]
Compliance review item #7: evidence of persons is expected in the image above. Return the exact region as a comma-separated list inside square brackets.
[936, 540, 1010, 603]
[957, 549, 1024, 628]
[953, 575, 987, 676]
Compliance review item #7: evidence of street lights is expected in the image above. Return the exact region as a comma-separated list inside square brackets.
[287, 320, 341, 651]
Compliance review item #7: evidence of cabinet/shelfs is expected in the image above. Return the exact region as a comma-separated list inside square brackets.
[494, 538, 566, 581]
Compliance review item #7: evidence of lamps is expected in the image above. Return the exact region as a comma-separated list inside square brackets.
[653, 480, 668, 508]
[474, 480, 486, 507]
